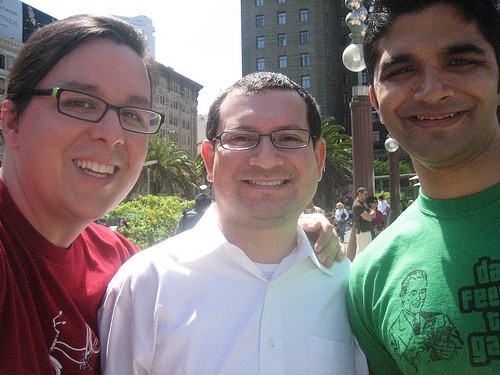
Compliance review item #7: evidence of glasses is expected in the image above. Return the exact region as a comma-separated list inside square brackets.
[6, 87, 165, 134]
[212, 129, 317, 151]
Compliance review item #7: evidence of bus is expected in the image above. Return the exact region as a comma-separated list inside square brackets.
[374, 173, 418, 203]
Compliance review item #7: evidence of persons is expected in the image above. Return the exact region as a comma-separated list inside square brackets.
[352, 187, 375, 254]
[378, 195, 390, 224]
[24, 5, 41, 41]
[176, 193, 211, 234]
[347, 0, 500, 375]
[1, 13, 344, 374]
[97, 72, 369, 375]
[368, 197, 385, 239]
[303, 201, 353, 242]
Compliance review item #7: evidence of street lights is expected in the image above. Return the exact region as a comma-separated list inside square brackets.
[382, 138, 403, 224]
[341, 0, 376, 205]
